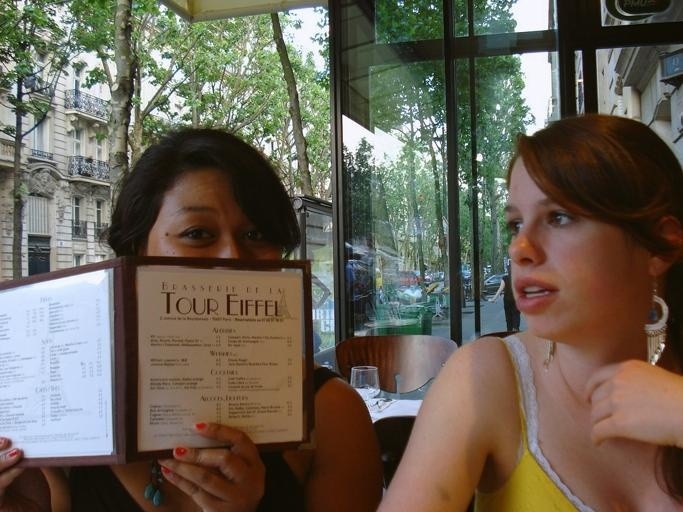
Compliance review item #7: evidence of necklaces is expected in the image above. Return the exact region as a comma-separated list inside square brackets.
[144, 456, 167, 507]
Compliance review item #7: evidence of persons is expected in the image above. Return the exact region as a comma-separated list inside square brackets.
[375, 114, 683, 512]
[0, 129, 383, 512]
[489, 266, 520, 332]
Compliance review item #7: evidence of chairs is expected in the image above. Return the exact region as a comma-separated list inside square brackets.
[335, 335, 459, 400]
[372, 416, 475, 512]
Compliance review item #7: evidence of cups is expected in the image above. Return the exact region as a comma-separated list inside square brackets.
[318, 364, 335, 373]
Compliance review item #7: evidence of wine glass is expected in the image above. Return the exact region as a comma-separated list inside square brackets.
[349, 365, 379, 412]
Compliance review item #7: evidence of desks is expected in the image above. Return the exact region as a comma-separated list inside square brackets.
[362, 398, 423, 423]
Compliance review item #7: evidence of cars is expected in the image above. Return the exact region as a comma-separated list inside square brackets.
[375, 252, 511, 305]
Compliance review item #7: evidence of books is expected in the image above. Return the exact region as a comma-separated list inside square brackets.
[0, 254, 314, 469]
